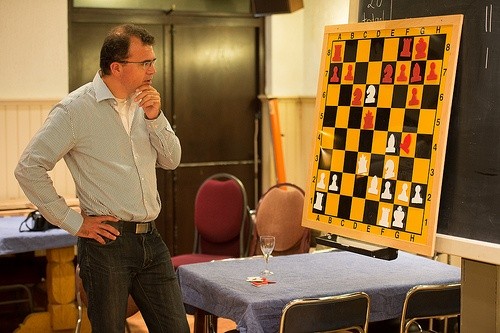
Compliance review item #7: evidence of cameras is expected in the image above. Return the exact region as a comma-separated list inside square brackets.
[30, 212, 56, 231]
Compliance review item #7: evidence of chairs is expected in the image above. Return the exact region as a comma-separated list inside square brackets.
[279, 293, 371, 333]
[400, 283, 461, 333]
[74, 259, 139, 333]
[244, 183, 312, 257]
[170, 175, 249, 271]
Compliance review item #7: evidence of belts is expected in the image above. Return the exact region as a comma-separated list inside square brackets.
[102, 218, 156, 234]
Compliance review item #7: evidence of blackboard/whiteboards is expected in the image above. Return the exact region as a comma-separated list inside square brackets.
[353, 1, 499, 251]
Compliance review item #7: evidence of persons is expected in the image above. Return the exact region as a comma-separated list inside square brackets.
[14, 24, 190, 333]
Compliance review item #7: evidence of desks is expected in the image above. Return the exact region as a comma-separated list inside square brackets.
[177, 248, 462, 333]
[0, 216, 83, 333]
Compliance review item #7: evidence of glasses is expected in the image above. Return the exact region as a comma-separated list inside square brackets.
[118, 59, 156, 70]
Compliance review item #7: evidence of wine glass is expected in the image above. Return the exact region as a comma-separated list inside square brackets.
[260, 236, 275, 276]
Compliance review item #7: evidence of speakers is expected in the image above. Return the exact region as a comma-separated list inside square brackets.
[252, 0, 304, 15]
